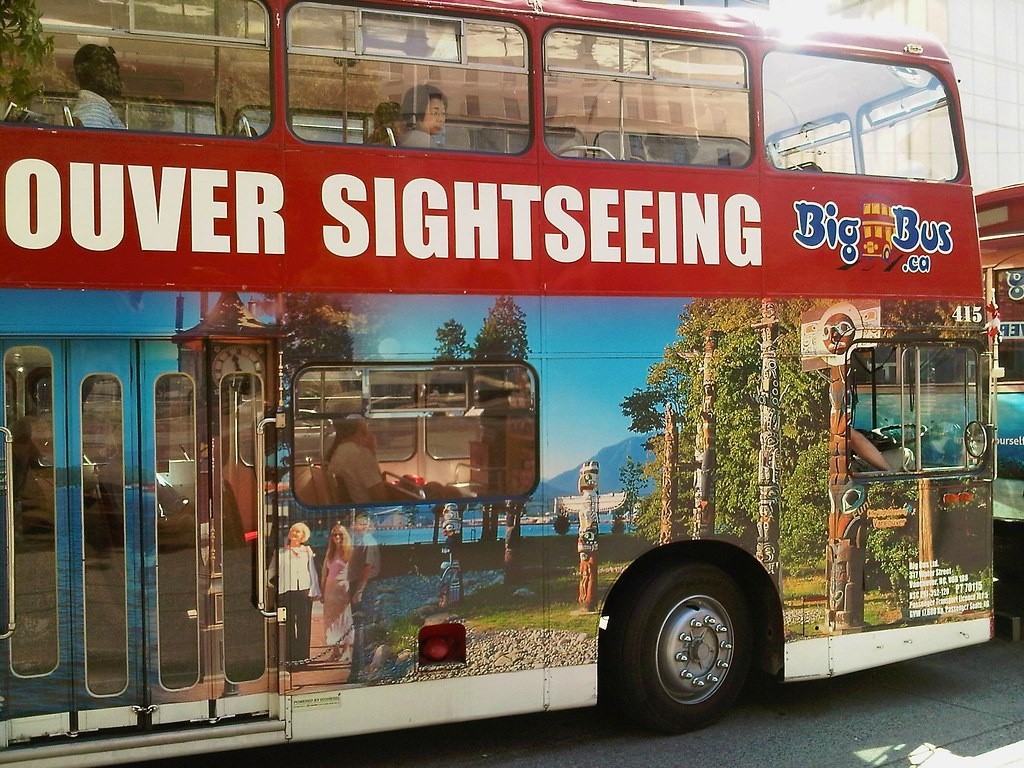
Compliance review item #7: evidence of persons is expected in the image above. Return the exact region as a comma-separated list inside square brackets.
[373, 102, 401, 135]
[71, 43, 126, 129]
[265, 522, 323, 673]
[348, 513, 381, 682]
[850, 427, 916, 472]
[327, 393, 385, 504]
[7, 417, 196, 594]
[397, 84, 449, 149]
[320, 525, 355, 662]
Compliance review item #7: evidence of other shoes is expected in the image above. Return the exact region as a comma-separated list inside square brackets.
[326, 653, 338, 661]
[336, 652, 351, 663]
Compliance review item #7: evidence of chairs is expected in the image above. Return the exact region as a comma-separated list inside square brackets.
[554, 145, 616, 161]
[309, 462, 342, 505]
[63, 105, 74, 127]
[383, 128, 397, 147]
[381, 470, 426, 503]
[788, 161, 820, 172]
[234, 112, 253, 138]
[446, 463, 489, 501]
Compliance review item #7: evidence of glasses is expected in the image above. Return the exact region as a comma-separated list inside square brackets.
[427, 110, 447, 118]
[331, 533, 342, 537]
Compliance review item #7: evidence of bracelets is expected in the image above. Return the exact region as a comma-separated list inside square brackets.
[356, 590, 363, 593]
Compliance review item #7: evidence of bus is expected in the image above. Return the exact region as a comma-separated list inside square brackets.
[0, 2, 996, 768]
